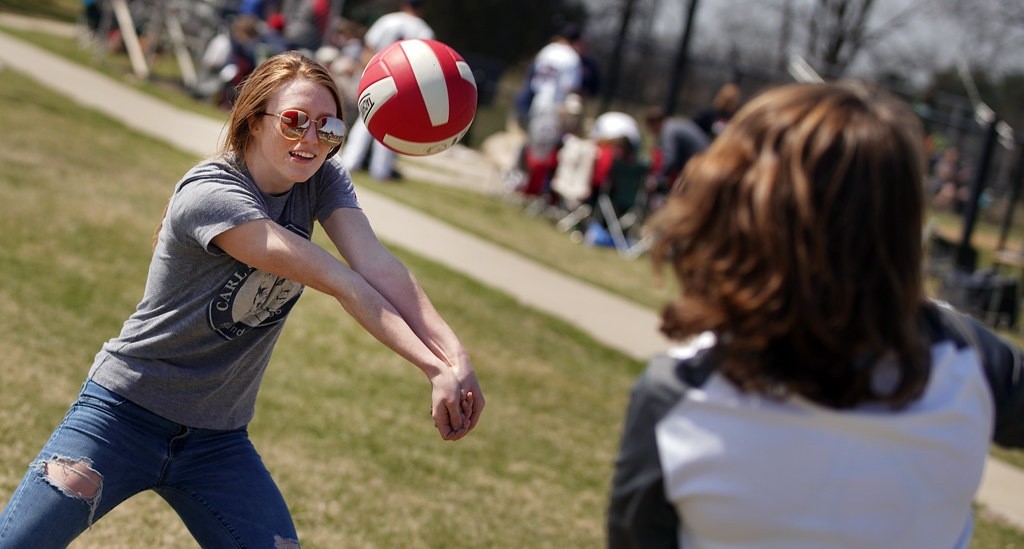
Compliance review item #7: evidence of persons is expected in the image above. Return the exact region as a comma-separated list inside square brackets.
[0, 54, 484, 549]
[601, 83, 1024, 549]
[80, 0, 976, 288]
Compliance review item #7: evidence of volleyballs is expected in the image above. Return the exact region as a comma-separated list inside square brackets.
[357, 37, 479, 157]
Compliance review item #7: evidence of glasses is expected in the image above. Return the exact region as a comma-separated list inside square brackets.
[262, 109, 347, 147]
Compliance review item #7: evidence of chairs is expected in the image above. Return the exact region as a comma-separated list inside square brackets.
[527, 133, 675, 267]
